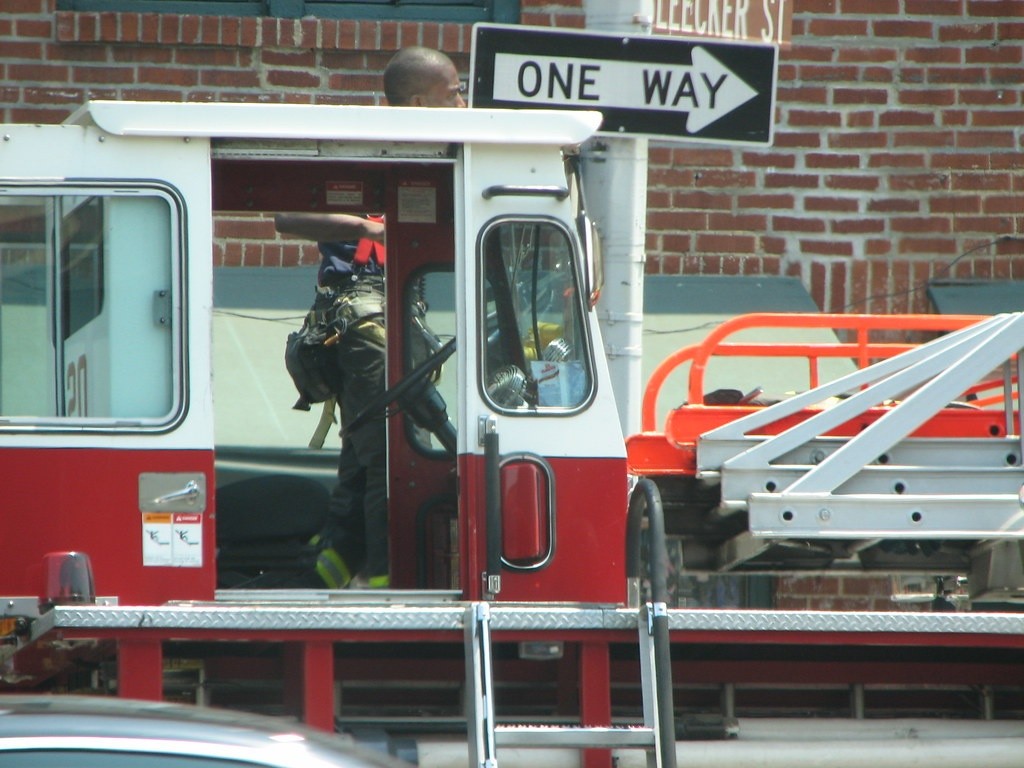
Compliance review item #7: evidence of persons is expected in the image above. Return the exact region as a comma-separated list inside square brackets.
[274, 45, 465, 590]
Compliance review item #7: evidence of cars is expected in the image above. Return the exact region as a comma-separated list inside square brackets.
[0, 694, 419, 768]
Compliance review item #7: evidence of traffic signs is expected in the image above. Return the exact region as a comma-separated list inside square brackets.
[468, 21, 780, 152]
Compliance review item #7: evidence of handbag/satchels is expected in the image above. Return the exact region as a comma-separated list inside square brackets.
[284, 328, 343, 403]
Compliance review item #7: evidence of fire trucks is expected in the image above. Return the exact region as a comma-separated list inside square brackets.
[1, 87, 1024, 768]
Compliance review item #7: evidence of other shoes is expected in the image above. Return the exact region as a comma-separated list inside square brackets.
[368, 574, 390, 588]
[316, 548, 350, 588]
